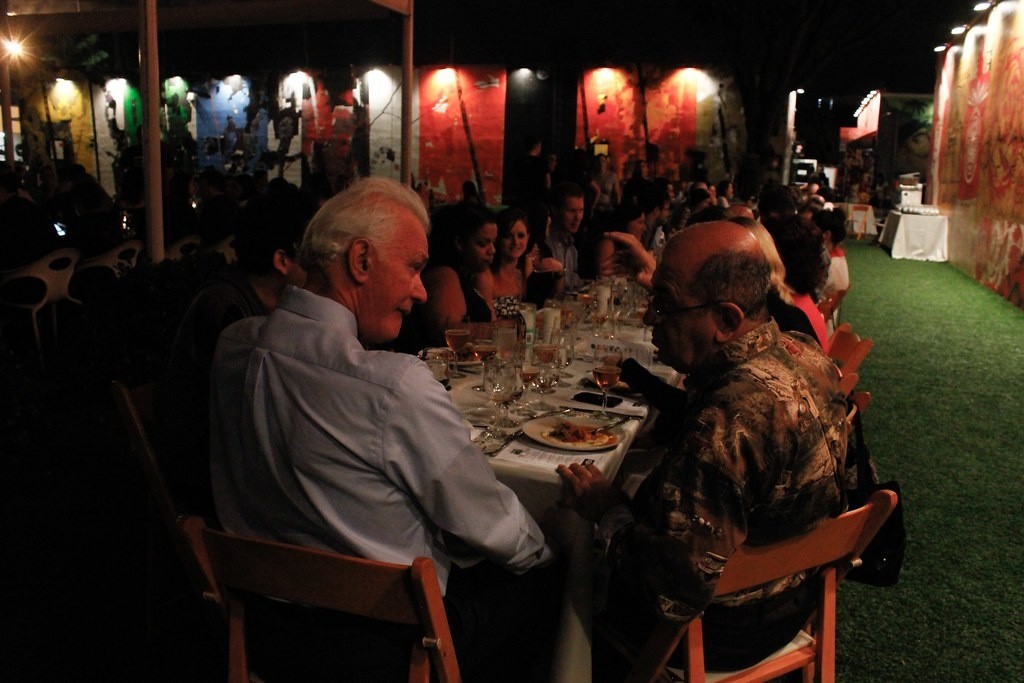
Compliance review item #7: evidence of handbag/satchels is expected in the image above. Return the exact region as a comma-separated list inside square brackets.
[839, 397, 907, 588]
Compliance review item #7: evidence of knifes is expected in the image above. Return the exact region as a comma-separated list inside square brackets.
[488, 428, 524, 457]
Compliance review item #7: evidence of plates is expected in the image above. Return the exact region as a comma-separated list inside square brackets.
[522, 416, 626, 451]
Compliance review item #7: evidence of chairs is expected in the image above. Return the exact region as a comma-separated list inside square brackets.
[180, 513, 463, 683]
[0, 247, 81, 353]
[74, 239, 143, 281]
[645, 488, 899, 683]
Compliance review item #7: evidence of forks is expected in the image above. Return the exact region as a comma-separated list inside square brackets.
[592, 416, 637, 433]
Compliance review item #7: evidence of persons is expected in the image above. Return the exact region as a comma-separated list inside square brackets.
[0, 136, 888, 683]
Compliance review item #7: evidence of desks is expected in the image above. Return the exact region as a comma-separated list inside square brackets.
[879, 209, 948, 262]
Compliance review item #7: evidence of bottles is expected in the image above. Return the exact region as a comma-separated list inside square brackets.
[569, 292, 586, 332]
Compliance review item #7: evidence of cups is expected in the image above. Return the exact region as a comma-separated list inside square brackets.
[597, 277, 612, 316]
[543, 297, 561, 345]
[520, 300, 535, 361]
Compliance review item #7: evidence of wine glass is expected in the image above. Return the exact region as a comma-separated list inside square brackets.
[592, 347, 623, 417]
[417, 316, 581, 446]
[586, 293, 642, 344]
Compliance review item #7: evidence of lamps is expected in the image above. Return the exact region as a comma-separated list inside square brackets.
[934, 45, 948, 53]
[974, 1, 991, 12]
[853, 90, 878, 118]
[950, 25, 966, 36]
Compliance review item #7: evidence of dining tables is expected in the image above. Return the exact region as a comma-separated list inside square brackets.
[412, 271, 681, 492]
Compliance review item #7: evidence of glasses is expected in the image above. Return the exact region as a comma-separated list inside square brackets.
[645, 290, 722, 320]
